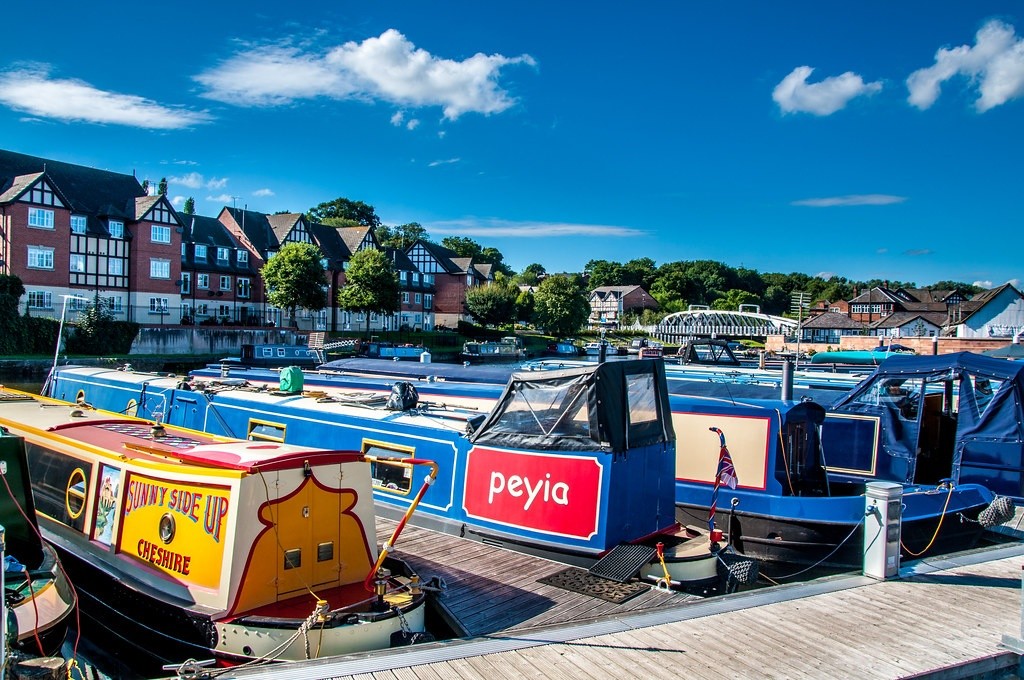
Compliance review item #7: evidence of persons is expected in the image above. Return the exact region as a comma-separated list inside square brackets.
[743, 347, 777, 360]
[678, 344, 686, 355]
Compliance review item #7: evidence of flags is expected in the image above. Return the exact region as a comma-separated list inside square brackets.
[721, 447, 738, 490]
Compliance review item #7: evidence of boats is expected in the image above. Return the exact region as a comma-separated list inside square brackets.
[1, 335, 1024, 680]
